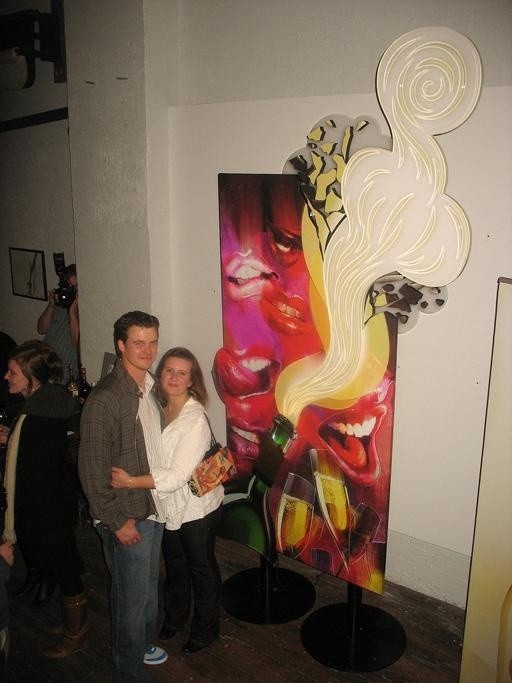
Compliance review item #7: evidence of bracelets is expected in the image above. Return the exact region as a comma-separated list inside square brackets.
[124, 476, 133, 491]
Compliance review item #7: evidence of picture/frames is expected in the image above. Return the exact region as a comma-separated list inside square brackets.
[7, 245, 49, 301]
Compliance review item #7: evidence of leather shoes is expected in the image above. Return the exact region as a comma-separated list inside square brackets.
[157, 621, 220, 654]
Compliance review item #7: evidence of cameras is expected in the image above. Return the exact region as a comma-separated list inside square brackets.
[52, 251, 76, 307]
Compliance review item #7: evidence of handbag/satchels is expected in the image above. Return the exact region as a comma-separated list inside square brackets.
[188, 411, 237, 499]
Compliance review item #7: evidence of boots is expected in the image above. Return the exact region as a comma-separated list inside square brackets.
[37, 593, 94, 659]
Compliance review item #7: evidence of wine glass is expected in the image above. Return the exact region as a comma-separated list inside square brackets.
[274, 445, 387, 596]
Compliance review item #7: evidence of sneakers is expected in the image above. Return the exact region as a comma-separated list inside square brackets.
[142, 645, 169, 667]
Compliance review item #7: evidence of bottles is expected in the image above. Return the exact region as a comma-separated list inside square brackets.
[206, 413, 297, 564]
[65, 362, 92, 407]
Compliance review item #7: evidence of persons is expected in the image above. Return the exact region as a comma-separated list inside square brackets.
[4, 340, 93, 660]
[0, 544, 14, 630]
[109, 347, 225, 656]
[34, 265, 81, 385]
[79, 310, 170, 683]
